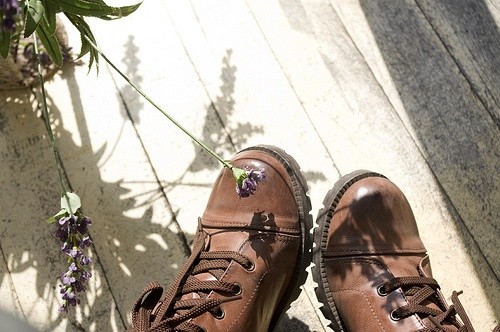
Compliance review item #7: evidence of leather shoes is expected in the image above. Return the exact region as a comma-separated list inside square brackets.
[132, 143, 315, 332]
[311, 168, 478, 332]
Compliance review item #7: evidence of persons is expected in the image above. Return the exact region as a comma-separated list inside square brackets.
[127, 144, 480, 332]
